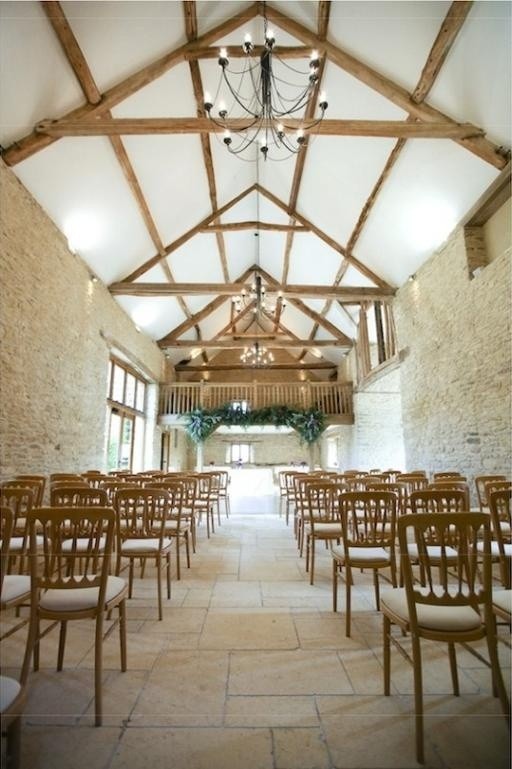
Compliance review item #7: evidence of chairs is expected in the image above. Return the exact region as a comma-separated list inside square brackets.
[1, 468, 229, 768]
[279, 469, 512, 769]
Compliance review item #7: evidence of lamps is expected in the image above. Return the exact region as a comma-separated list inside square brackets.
[238, 331, 274, 367]
[230, 234, 285, 322]
[204, 1, 327, 163]
